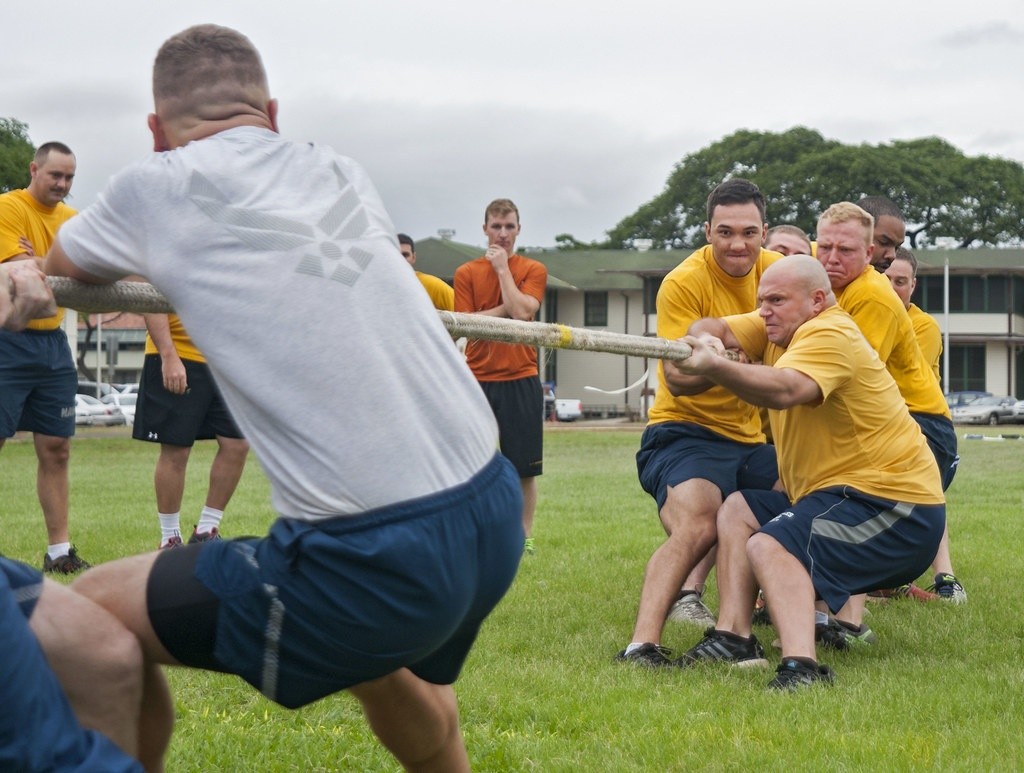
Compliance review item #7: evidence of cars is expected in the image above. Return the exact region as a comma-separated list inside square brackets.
[942, 390, 1024, 426]
[542, 388, 582, 423]
[100, 392, 138, 425]
[74, 394, 126, 427]
[76, 380, 139, 400]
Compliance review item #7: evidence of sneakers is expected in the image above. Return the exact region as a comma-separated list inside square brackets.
[925, 572, 967, 606]
[868, 582, 935, 601]
[752, 592, 771, 625]
[43, 545, 92, 575]
[816, 619, 876, 650]
[668, 584, 716, 627]
[675, 626, 769, 667]
[615, 643, 673, 667]
[766, 656, 832, 689]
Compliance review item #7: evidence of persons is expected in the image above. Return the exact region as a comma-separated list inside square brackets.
[614, 180, 968, 669]
[396, 232, 456, 311]
[0, 141, 92, 574]
[671, 253, 948, 690]
[455, 198, 548, 556]
[0, 24, 526, 773]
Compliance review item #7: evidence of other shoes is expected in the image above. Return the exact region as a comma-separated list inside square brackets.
[523, 537, 535, 555]
[188, 524, 223, 543]
[158, 530, 184, 550]
